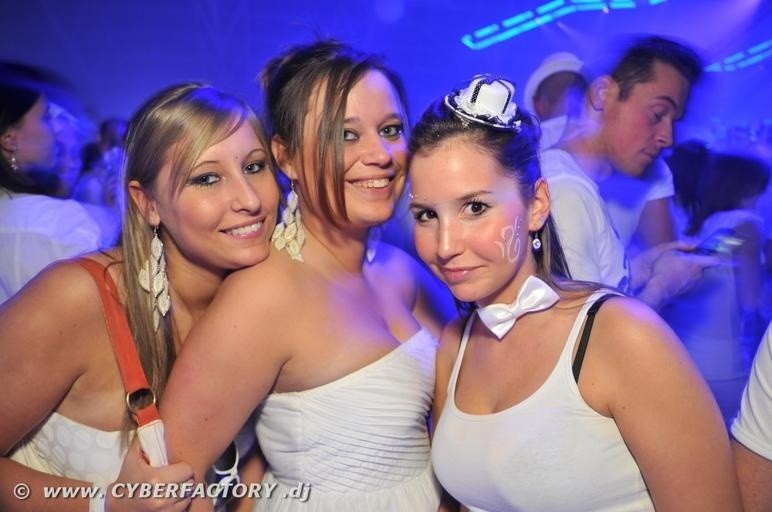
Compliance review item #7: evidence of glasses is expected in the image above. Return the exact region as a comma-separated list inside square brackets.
[211, 440, 239, 511]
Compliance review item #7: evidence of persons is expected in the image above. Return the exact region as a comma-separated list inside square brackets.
[0, 34, 772, 512]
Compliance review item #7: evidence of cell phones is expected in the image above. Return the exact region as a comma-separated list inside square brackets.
[692, 228, 736, 256]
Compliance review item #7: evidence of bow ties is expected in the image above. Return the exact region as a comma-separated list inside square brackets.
[476, 275, 559, 341]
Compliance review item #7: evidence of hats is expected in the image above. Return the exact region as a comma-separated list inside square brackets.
[444, 72, 522, 132]
[522, 51, 593, 125]
